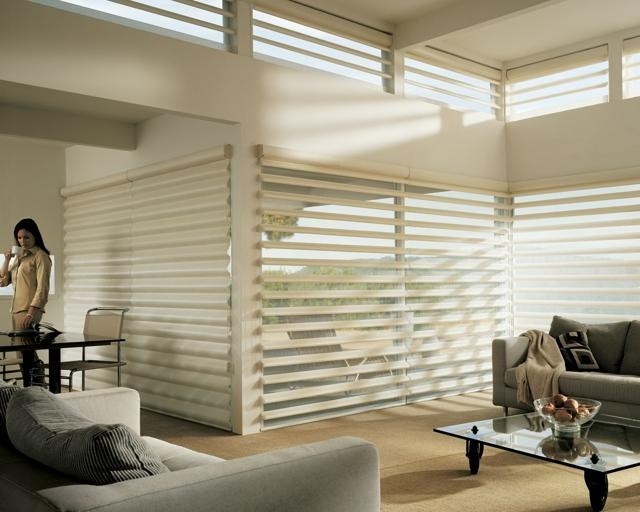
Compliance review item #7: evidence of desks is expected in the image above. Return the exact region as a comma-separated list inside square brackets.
[0, 326, 126, 394]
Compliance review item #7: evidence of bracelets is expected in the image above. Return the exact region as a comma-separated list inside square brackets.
[27, 313, 34, 319]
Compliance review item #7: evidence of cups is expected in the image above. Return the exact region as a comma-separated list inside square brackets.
[11, 245, 22, 255]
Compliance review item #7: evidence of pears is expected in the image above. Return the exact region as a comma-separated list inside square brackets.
[542, 394, 589, 422]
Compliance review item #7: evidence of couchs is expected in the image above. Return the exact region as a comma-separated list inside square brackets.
[0, 384, 381, 512]
[492, 316, 640, 424]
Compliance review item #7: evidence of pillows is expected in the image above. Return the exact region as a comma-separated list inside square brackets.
[553, 329, 600, 371]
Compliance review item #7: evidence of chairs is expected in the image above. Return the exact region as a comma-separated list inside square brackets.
[29, 307, 130, 392]
[277, 309, 393, 397]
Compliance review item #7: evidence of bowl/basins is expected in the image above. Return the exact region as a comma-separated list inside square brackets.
[533, 396, 602, 433]
[533, 433, 605, 467]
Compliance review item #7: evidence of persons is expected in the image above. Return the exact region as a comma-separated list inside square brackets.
[0, 218, 52, 391]
